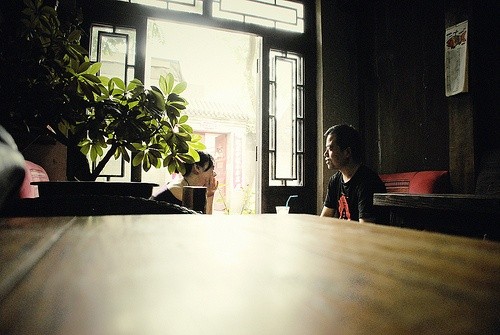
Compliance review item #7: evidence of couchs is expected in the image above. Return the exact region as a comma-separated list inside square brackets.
[377, 169, 451, 193]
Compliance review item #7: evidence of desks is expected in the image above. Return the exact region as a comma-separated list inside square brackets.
[372, 192, 500, 243]
[0, 210, 500, 334]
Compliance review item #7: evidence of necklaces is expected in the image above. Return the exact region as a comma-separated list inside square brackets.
[184, 177, 190, 187]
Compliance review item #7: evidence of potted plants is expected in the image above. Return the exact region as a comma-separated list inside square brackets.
[4, 0, 208, 202]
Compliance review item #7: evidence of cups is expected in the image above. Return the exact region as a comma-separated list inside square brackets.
[182, 185, 208, 214]
[276, 206, 290, 214]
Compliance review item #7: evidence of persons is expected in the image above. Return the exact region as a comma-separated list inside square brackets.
[319, 124, 390, 227]
[152, 150, 219, 215]
